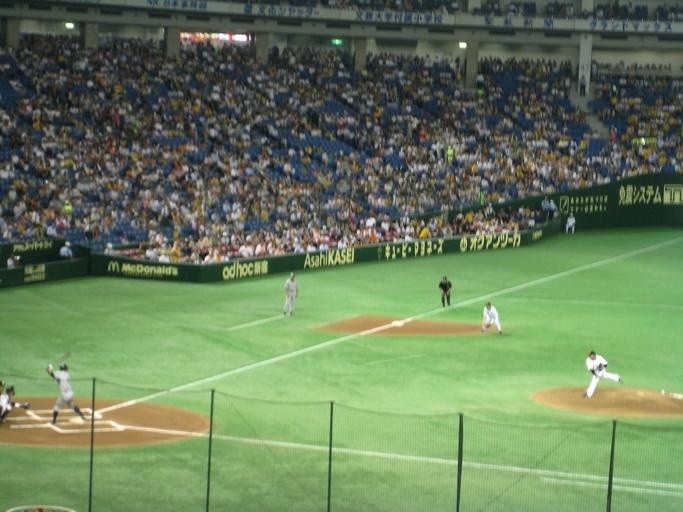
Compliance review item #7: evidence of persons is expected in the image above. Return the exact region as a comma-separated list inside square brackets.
[438, 275, 452, 307]
[282, 271, 298, 316]
[44, 362, 85, 425]
[0, 384, 27, 424]
[480, 301, 503, 335]
[582, 350, 623, 399]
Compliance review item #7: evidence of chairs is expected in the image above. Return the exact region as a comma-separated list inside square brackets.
[0, 33, 683, 257]
